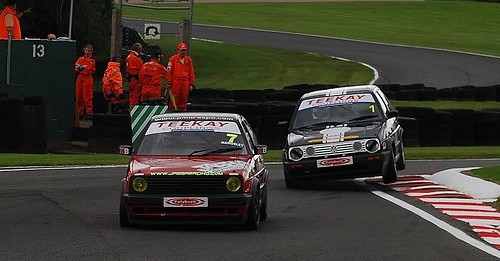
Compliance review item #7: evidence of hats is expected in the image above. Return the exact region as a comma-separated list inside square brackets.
[178, 43, 187, 50]
[152, 53, 163, 58]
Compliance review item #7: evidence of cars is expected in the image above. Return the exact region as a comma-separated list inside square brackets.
[277, 84, 406, 186]
[117, 112, 270, 231]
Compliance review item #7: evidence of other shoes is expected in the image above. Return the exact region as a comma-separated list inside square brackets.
[85, 115, 92, 120]
[79, 115, 83, 120]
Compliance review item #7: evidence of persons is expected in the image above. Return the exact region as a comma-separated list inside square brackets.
[160, 131, 182, 145]
[126, 43, 144, 112]
[303, 106, 330, 124]
[75, 46, 96, 120]
[0, 0, 32, 39]
[139, 54, 169, 104]
[166, 43, 195, 112]
[102, 60, 123, 113]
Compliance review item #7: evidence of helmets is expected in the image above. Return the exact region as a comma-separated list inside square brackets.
[312, 107, 331, 120]
[161, 132, 183, 146]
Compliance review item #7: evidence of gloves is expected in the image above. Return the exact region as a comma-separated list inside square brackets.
[79, 66, 84, 71]
